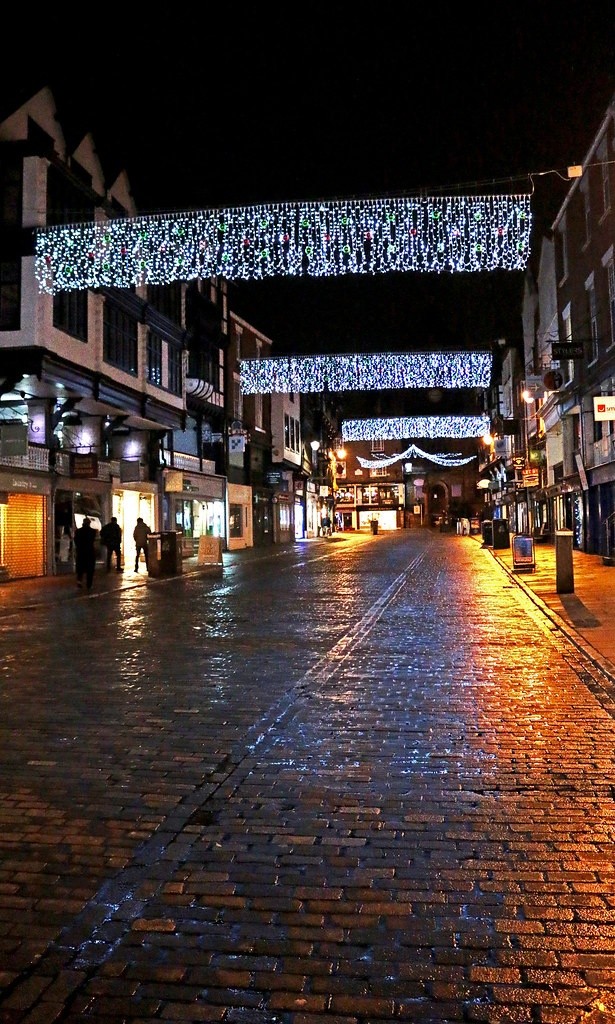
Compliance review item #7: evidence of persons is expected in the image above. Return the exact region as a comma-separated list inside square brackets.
[133, 518, 151, 573]
[99, 517, 126, 571]
[73, 518, 98, 592]
[321, 513, 331, 537]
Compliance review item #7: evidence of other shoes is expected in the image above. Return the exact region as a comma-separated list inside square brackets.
[134, 570, 138, 572]
[117, 568, 124, 571]
[107, 567, 111, 570]
[87, 588, 91, 593]
[77, 584, 82, 588]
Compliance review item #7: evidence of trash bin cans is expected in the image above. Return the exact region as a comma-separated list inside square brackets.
[492, 518, 510, 549]
[146, 531, 176, 577]
[481, 521, 492, 545]
